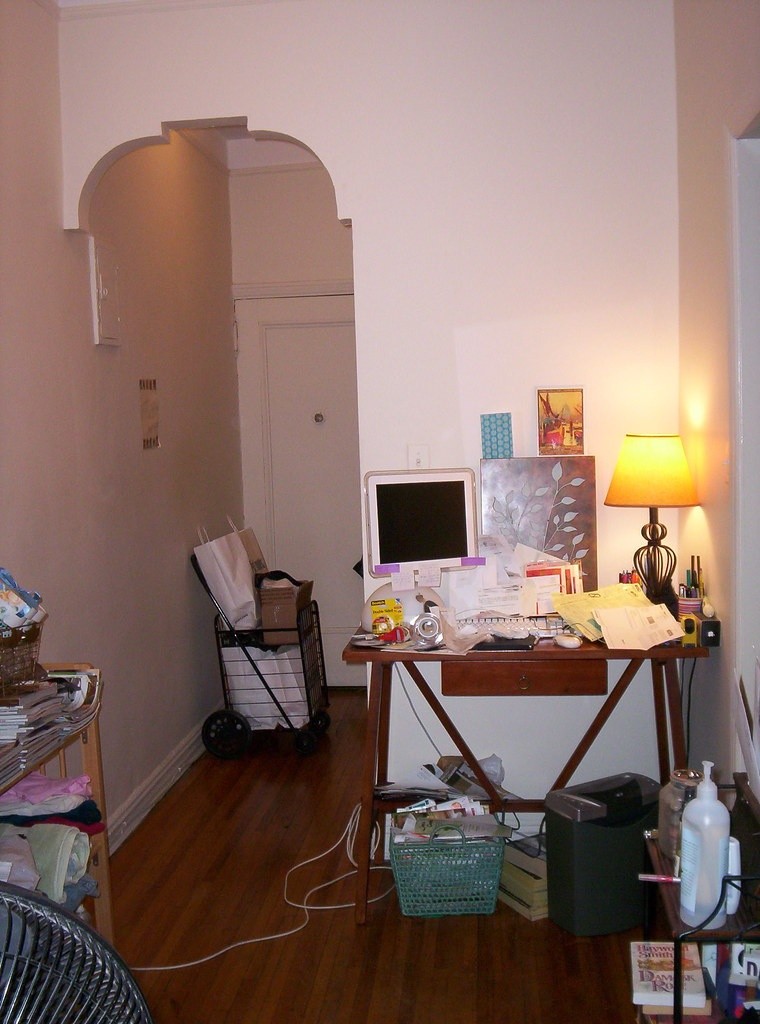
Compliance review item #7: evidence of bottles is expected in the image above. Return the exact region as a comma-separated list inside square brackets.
[660, 769, 705, 858]
[681, 761, 730, 930]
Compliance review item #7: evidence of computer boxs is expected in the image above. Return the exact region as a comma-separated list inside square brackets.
[545, 771, 664, 937]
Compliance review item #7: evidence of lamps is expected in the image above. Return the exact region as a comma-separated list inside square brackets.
[603, 433, 703, 606]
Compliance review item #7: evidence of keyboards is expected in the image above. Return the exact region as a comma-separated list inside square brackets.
[457, 617, 583, 641]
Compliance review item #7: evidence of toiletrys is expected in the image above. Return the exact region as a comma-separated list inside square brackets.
[678, 760, 742, 931]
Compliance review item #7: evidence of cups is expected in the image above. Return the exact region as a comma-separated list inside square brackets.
[678, 597, 701, 621]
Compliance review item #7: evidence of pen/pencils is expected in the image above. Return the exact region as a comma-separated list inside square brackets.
[678, 555, 704, 598]
[636, 873, 683, 884]
[619, 566, 642, 585]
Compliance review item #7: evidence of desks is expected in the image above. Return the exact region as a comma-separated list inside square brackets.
[342, 625, 710, 926]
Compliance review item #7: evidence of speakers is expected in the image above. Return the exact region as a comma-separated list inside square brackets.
[413, 612, 446, 651]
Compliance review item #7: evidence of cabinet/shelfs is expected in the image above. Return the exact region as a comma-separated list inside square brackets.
[643, 828, 760, 1024]
[0, 663, 115, 951]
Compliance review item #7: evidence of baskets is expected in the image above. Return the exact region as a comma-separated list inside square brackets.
[389, 814, 506, 917]
[0, 625, 42, 696]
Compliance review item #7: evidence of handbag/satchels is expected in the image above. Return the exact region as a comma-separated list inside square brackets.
[193, 512, 270, 632]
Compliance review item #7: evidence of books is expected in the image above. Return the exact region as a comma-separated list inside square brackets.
[498, 833, 549, 920]
[524, 561, 582, 637]
[631, 941, 714, 1015]
[389, 796, 513, 895]
[0, 669, 102, 789]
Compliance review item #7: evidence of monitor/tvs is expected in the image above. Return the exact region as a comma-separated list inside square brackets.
[361, 468, 479, 632]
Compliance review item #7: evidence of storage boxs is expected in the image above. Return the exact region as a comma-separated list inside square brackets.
[678, 597, 702, 623]
[692, 611, 721, 647]
[0, 622, 44, 693]
[256, 580, 314, 644]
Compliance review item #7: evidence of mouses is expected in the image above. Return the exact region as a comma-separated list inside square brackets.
[552, 634, 583, 648]
